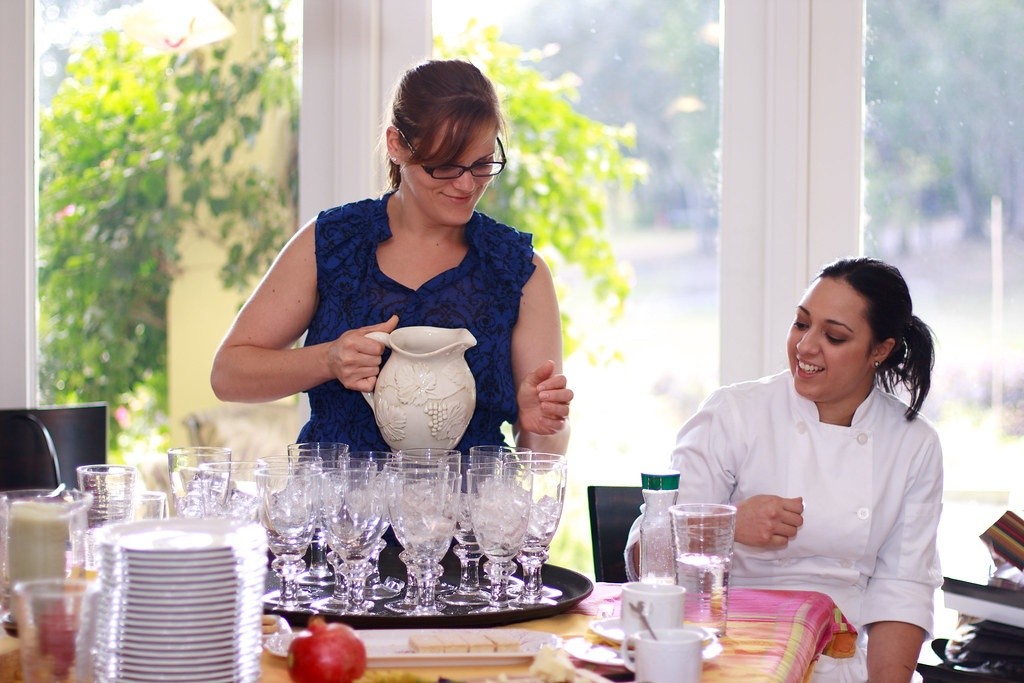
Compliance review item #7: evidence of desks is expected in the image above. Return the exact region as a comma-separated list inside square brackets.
[0, 582, 858, 682]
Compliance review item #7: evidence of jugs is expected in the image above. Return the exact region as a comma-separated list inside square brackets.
[358, 326, 477, 455]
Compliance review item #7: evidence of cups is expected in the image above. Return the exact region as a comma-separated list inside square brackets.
[668, 503, 737, 635]
[620, 581, 686, 633]
[78, 464, 137, 527]
[14, 578, 101, 683]
[1, 489, 93, 632]
[621, 628, 704, 683]
[109, 490, 166, 523]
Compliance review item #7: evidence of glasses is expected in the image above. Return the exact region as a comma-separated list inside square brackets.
[395, 126, 507, 180]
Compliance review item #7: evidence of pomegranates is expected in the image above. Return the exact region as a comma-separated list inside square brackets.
[286, 615, 367, 683]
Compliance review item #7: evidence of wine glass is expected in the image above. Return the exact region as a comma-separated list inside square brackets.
[167, 441, 568, 616]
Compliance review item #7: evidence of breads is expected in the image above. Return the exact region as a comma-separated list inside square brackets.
[408, 633, 520, 653]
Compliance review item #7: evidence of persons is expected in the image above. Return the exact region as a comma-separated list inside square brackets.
[210, 59, 573, 546]
[623, 258, 944, 683]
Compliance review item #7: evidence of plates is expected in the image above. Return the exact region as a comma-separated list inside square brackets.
[565, 637, 723, 666]
[263, 628, 562, 668]
[83, 517, 269, 683]
[588, 616, 712, 644]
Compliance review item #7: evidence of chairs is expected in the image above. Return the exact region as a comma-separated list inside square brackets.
[588, 485, 645, 582]
[0, 404, 108, 493]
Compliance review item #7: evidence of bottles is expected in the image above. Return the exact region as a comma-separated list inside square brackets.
[638, 468, 681, 583]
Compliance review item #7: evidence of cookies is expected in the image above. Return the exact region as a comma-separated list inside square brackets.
[262, 616, 278, 634]
